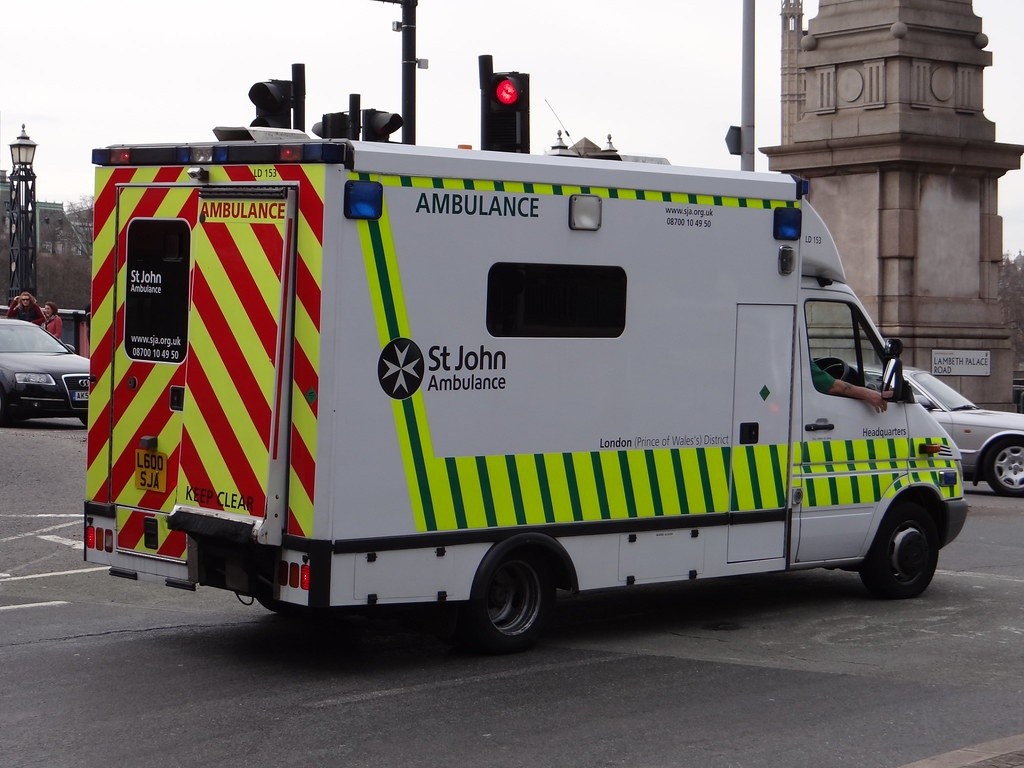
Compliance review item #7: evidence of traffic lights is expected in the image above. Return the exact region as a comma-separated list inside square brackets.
[248, 75, 296, 131]
[362, 108, 405, 142]
[312, 111, 350, 141]
[483, 71, 530, 154]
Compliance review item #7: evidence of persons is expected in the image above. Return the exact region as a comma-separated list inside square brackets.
[42, 301, 62, 339]
[6, 292, 46, 325]
[807, 359, 887, 412]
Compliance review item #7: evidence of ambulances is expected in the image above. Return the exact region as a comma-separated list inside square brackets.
[81, 124, 971, 658]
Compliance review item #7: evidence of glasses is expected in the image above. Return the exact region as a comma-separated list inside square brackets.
[21, 299, 29, 301]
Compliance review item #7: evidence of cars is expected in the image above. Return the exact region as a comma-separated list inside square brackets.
[847, 364, 1024, 497]
[1, 314, 90, 426]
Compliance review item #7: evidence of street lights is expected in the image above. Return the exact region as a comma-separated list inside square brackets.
[6, 124, 40, 306]
